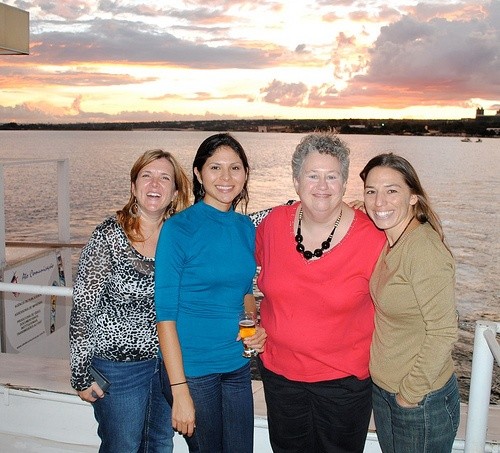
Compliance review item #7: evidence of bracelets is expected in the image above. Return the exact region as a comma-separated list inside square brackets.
[170, 381, 187, 387]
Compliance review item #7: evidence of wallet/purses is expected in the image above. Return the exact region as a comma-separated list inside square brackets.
[88, 364, 111, 399]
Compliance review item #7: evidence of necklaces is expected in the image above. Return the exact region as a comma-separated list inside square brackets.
[389, 216, 415, 249]
[295, 207, 343, 260]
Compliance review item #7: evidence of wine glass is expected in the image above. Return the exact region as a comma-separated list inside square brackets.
[239, 312, 258, 358]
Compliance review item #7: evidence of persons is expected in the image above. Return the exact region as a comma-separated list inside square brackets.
[252, 133, 388, 453]
[69, 149, 297, 453]
[347, 153, 461, 453]
[155, 133, 256, 453]
